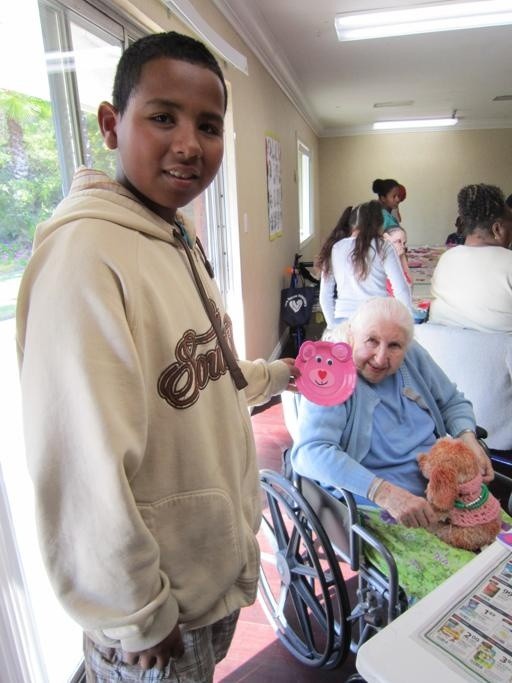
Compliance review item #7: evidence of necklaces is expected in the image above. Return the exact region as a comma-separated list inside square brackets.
[454, 483, 488, 511]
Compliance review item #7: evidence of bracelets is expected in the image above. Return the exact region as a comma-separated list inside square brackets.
[458, 429, 476, 437]
[369, 478, 386, 501]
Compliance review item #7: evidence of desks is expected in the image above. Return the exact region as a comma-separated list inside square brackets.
[356, 529, 512, 683]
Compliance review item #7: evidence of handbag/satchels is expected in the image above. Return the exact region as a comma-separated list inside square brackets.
[279, 269, 316, 328]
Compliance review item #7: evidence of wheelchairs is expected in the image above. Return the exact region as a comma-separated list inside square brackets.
[251, 424, 512, 673]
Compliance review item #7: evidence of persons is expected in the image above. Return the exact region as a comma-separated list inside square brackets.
[316, 177, 512, 333]
[288, 299, 497, 529]
[11, 29, 301, 683]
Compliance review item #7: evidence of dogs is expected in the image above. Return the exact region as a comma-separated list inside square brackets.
[415, 434, 505, 551]
